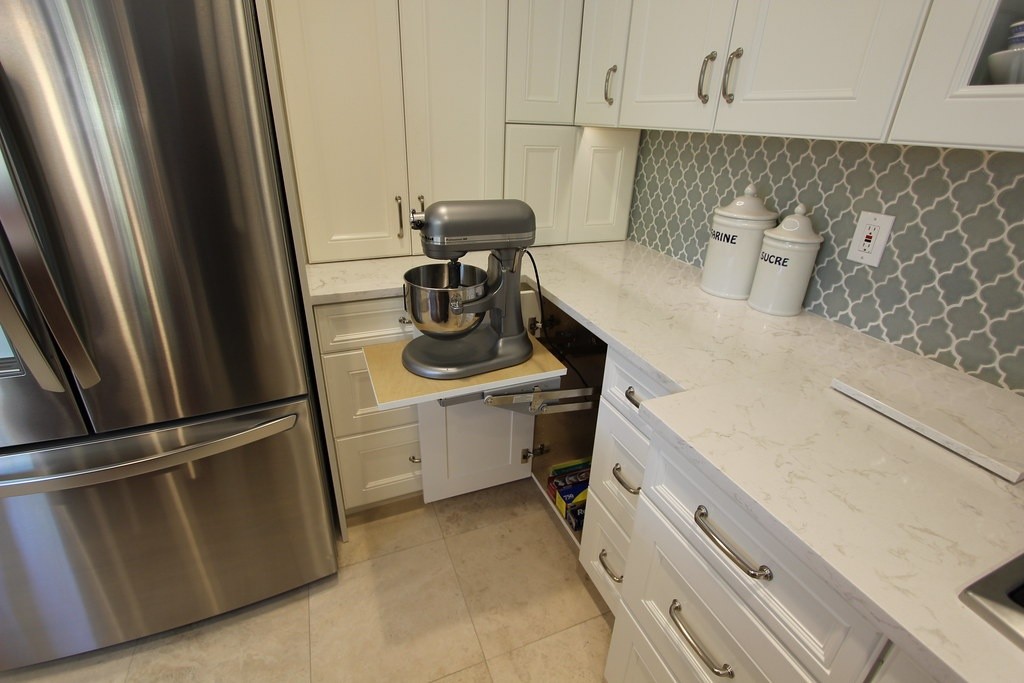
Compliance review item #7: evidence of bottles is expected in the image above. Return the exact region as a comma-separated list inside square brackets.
[699, 183, 780, 300]
[746, 202, 824, 316]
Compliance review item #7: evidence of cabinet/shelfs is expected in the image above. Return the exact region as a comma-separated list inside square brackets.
[414, 290, 607, 550]
[255, 0, 508, 264]
[504, 0, 641, 246]
[312, 296, 425, 511]
[604, 429, 940, 683]
[573, 0, 1024, 152]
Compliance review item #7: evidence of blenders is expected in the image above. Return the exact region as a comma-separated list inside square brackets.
[401, 198, 536, 381]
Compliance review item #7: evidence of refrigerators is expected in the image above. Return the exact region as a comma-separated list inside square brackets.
[0, 1, 341, 672]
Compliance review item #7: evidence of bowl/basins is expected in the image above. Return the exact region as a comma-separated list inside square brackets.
[986, 20, 1024, 84]
